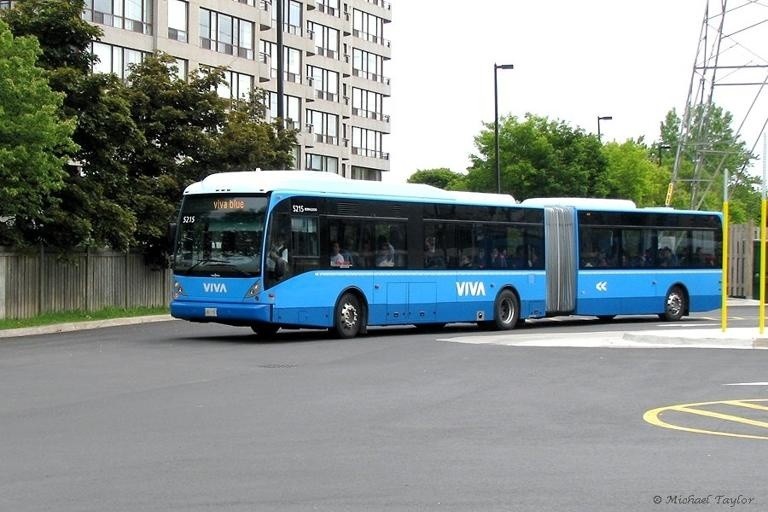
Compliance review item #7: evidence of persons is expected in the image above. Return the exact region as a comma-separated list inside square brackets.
[233, 227, 538, 278]
[578, 241, 715, 268]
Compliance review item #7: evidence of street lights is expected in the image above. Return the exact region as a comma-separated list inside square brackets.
[492, 64, 514, 193]
[597, 116, 613, 142]
[658, 145, 672, 166]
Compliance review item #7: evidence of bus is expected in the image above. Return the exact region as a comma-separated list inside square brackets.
[166, 167, 725, 341]
